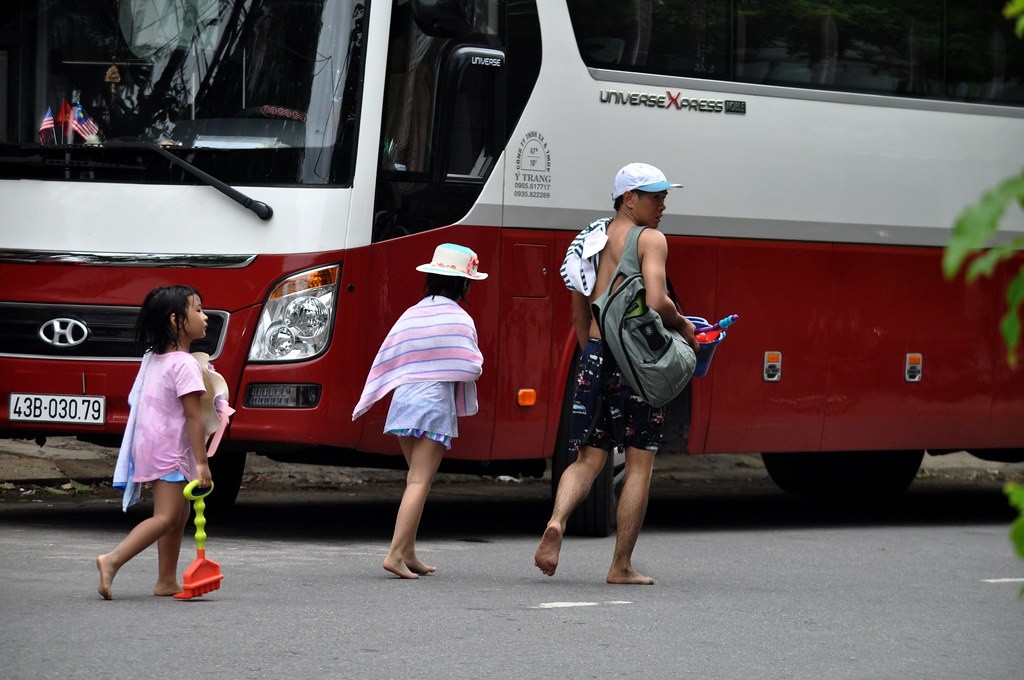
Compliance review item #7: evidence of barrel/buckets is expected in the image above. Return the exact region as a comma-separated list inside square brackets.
[680, 316, 724, 378]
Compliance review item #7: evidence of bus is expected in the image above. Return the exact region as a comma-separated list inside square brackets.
[0, 0, 1024, 540]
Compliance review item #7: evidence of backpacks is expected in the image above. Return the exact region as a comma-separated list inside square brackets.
[591, 225, 697, 408]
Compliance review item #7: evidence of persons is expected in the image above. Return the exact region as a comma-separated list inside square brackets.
[96, 284, 212, 600]
[534, 162, 700, 585]
[351, 242, 488, 579]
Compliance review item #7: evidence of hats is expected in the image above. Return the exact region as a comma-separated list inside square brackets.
[611, 162, 684, 200]
[416, 243, 488, 280]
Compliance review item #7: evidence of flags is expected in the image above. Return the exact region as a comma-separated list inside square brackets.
[72, 103, 99, 141]
[57, 100, 72, 135]
[38, 106, 54, 146]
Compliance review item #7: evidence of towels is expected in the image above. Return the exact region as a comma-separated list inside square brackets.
[351, 294, 485, 422]
[111, 344, 159, 512]
